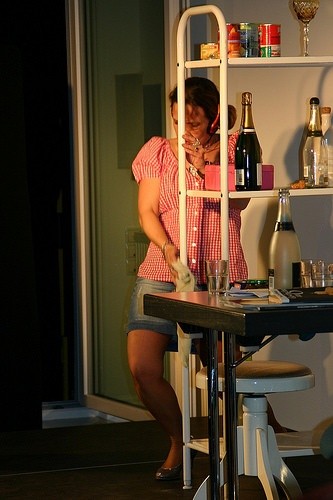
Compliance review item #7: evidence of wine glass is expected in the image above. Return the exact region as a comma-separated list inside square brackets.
[288, 0, 320, 57]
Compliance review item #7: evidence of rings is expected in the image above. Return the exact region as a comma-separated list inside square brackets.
[195, 147, 199, 152]
[194, 140, 201, 146]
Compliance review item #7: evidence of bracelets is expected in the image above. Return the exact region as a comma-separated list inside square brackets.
[197, 161, 213, 180]
[162, 242, 172, 253]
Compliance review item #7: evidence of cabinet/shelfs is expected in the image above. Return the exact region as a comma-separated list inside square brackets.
[177, 4, 333, 489]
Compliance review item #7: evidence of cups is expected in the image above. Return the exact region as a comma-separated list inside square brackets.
[239, 22, 259, 57]
[206, 259, 229, 298]
[300, 257, 312, 276]
[324, 263, 333, 280]
[312, 260, 324, 280]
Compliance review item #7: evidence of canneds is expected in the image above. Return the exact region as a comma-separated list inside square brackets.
[200, 22, 281, 60]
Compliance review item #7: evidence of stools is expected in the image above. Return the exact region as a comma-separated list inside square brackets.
[193, 360, 315, 500]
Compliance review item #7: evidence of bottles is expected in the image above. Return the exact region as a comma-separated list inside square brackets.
[268, 269, 275, 294]
[234, 91, 261, 191]
[299, 97, 328, 188]
[269, 187, 302, 290]
[320, 107, 333, 188]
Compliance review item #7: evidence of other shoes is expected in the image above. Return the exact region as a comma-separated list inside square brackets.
[154, 462, 183, 481]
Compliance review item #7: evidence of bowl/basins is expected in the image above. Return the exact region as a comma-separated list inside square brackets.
[230, 279, 269, 290]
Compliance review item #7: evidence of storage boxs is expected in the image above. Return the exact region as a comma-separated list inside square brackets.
[205, 164, 274, 191]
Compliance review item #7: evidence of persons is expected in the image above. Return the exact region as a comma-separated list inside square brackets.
[125, 76, 263, 480]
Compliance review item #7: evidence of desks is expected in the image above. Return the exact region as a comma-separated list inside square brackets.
[144, 290, 333, 500]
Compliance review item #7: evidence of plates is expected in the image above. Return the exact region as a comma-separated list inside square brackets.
[223, 289, 256, 297]
